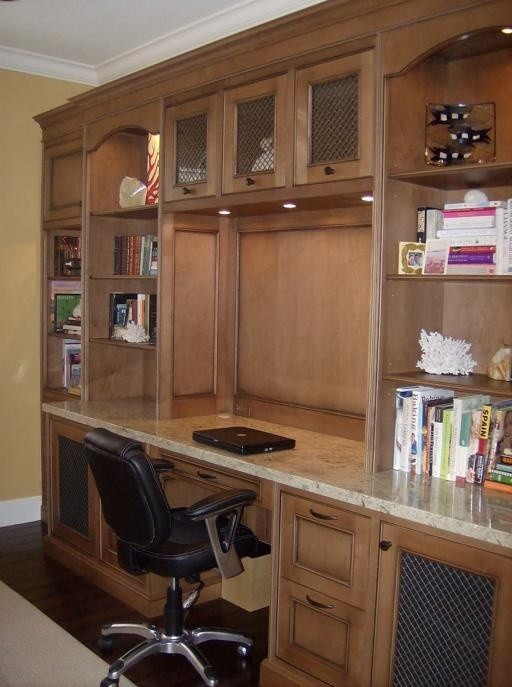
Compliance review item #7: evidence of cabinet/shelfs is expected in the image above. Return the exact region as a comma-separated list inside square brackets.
[84, 100, 223, 418]
[46, 414, 151, 598]
[268, 481, 376, 687]
[370, 517, 512, 687]
[366, 0, 512, 496]
[162, 35, 377, 203]
[41, 125, 84, 401]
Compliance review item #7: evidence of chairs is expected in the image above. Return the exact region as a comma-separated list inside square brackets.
[81, 426, 257, 687]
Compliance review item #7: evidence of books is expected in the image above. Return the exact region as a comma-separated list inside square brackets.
[59, 338, 81, 390]
[416, 198, 512, 276]
[47, 279, 82, 336]
[114, 234, 158, 276]
[108, 291, 156, 344]
[392, 385, 512, 495]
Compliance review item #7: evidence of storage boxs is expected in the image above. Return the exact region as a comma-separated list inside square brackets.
[222, 549, 269, 612]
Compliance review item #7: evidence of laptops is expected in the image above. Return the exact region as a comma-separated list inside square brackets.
[190, 425, 299, 456]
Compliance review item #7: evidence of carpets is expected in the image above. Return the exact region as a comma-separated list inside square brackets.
[0, 520, 268, 687]
